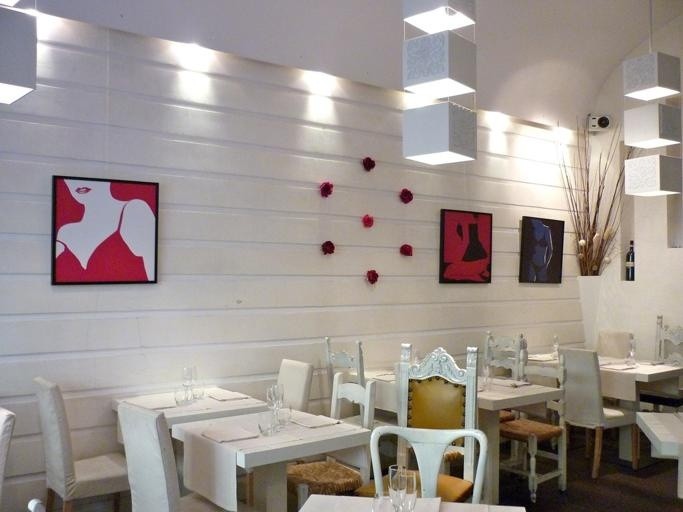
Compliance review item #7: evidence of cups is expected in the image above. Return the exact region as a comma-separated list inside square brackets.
[257, 383, 292, 436]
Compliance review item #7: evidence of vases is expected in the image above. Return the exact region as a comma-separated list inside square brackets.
[580, 275, 601, 350]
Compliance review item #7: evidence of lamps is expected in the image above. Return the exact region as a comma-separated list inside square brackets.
[403, 0, 477, 166]
[624, 1, 683, 197]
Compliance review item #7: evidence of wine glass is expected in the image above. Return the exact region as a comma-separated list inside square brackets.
[628, 339, 637, 364]
[551, 335, 560, 352]
[481, 359, 492, 392]
[174, 365, 205, 406]
[370, 465, 418, 512]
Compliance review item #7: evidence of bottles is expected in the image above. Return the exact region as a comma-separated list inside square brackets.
[626, 239, 634, 280]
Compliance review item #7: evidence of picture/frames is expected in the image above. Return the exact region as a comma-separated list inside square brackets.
[440, 209, 492, 283]
[520, 216, 564, 283]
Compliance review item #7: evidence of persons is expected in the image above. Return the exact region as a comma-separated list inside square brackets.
[529, 219, 553, 282]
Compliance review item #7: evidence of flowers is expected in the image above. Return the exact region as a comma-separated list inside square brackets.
[558, 112, 627, 276]
